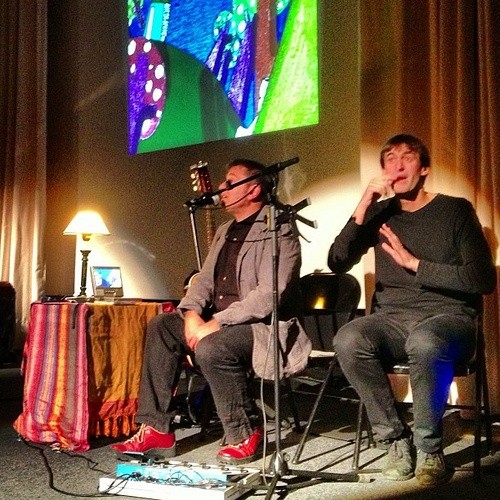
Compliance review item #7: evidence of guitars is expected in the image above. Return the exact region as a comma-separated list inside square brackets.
[183, 161, 217, 298]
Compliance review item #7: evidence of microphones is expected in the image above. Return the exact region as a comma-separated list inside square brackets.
[183, 195, 220, 208]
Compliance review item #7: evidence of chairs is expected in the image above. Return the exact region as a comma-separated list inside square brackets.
[279, 272, 379, 466]
[352, 284, 496, 488]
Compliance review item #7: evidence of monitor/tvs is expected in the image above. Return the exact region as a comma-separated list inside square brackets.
[90, 266, 123, 297]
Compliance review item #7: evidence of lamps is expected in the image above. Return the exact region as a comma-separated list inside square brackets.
[62, 209, 112, 303]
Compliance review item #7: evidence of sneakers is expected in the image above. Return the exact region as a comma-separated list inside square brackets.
[415, 447, 449, 488]
[382, 431, 414, 480]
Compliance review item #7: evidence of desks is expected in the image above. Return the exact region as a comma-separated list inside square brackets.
[29, 302, 176, 449]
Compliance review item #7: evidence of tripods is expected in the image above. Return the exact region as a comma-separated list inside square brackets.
[197, 158, 375, 500]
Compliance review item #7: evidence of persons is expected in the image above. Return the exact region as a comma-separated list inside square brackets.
[327, 134, 497, 487]
[109, 158, 313, 464]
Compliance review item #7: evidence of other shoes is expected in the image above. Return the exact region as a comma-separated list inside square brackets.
[106, 424, 177, 460]
[216, 428, 269, 465]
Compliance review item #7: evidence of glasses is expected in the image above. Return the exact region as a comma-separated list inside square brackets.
[226, 180, 233, 189]
